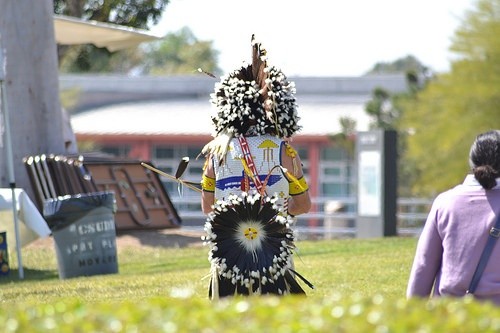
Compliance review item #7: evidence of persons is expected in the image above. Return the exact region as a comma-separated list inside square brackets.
[194, 33, 315, 299]
[405, 130, 500, 304]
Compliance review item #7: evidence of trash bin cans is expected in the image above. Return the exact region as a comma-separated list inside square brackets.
[41, 190, 118, 280]
